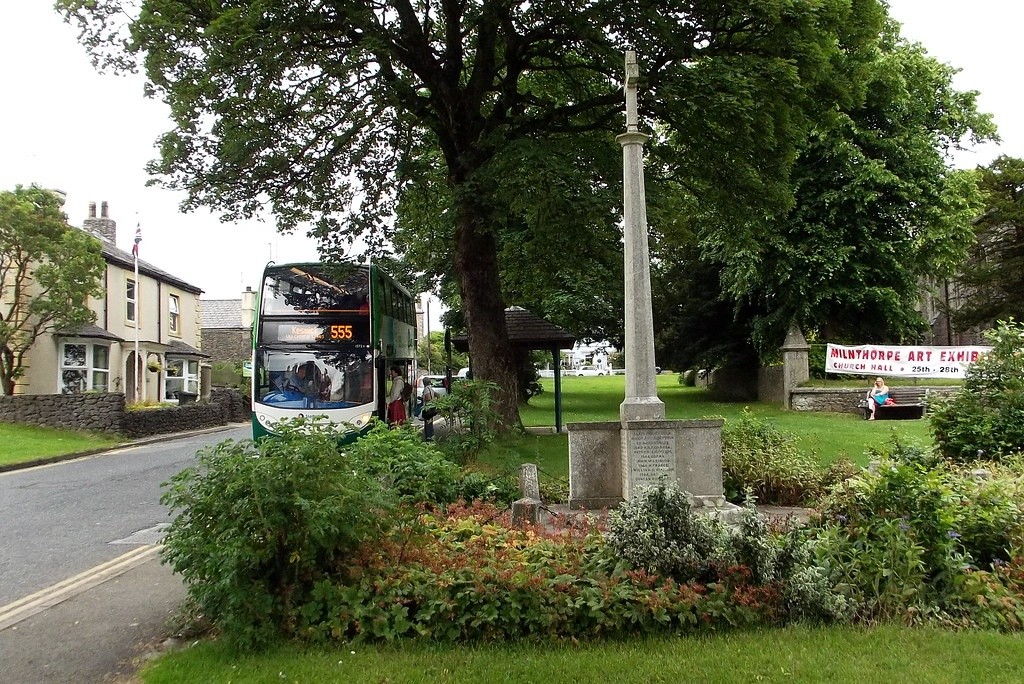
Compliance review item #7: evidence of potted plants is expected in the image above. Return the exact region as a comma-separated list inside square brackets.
[148, 360, 162, 372]
[166, 363, 178, 376]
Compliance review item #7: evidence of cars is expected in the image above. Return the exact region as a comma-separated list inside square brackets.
[575, 365, 607, 377]
[448, 368, 470, 386]
[415, 375, 450, 401]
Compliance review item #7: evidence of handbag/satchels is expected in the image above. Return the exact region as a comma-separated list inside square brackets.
[418, 405, 425, 421]
[885, 398, 895, 405]
[414, 399, 424, 417]
[422, 406, 437, 420]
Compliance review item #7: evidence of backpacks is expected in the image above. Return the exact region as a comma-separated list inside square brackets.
[394, 378, 413, 402]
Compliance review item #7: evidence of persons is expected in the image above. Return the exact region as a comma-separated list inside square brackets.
[356, 295, 369, 314]
[282, 364, 312, 394]
[866, 377, 891, 420]
[386, 366, 405, 426]
[417, 377, 440, 442]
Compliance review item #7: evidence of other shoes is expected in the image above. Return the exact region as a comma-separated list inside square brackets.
[868, 418, 875, 421]
[867, 409, 873, 414]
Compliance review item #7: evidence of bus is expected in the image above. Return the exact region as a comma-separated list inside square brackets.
[248, 259, 419, 454]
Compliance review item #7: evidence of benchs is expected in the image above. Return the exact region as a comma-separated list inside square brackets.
[857, 389, 930, 420]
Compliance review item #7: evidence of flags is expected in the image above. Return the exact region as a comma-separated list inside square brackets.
[131, 222, 142, 256]
